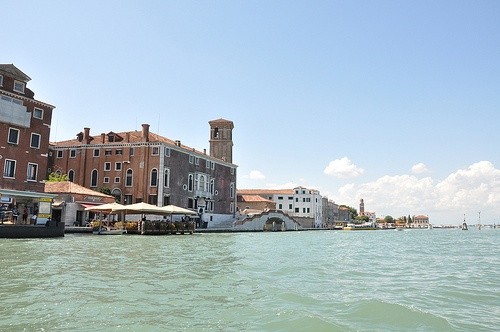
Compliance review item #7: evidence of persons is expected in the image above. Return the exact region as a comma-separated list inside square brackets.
[0, 204, 6, 224]
[182, 216, 187, 222]
[32, 203, 39, 225]
[162, 216, 167, 222]
[22, 205, 28, 225]
[142, 217, 146, 221]
[12, 205, 18, 225]
[184, 214, 189, 221]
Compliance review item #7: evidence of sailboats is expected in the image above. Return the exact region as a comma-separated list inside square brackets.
[462, 214, 468, 230]
[476, 212, 481, 230]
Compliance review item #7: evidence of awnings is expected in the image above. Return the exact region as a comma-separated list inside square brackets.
[81, 204, 96, 208]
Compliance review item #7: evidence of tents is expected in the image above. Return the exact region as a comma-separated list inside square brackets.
[113, 201, 158, 222]
[85, 202, 125, 226]
[142, 205, 198, 226]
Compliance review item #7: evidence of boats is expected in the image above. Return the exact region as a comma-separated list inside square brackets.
[396, 227, 403, 231]
